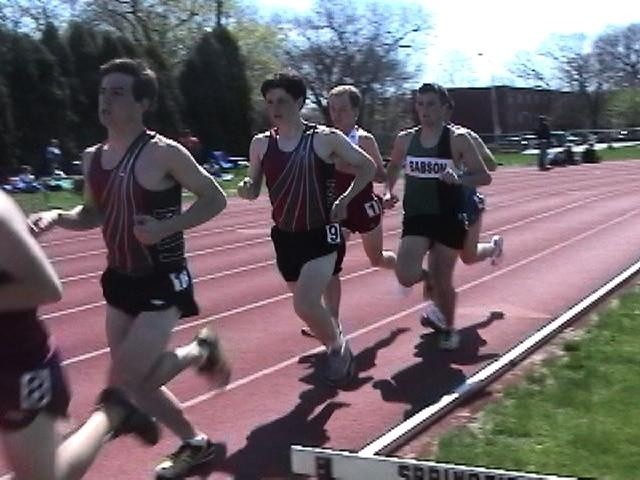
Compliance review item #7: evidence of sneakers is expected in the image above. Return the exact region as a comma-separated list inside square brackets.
[318, 337, 355, 387]
[420, 304, 460, 352]
[155, 433, 216, 478]
[301, 327, 342, 341]
[96, 387, 158, 446]
[491, 235, 503, 265]
[194, 327, 231, 386]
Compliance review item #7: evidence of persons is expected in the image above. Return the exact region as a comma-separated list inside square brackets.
[24, 59, 235, 479]
[537, 115, 554, 172]
[418, 97, 505, 328]
[0, 185, 162, 479]
[236, 72, 382, 390]
[545, 149, 567, 167]
[380, 80, 493, 354]
[300, 86, 400, 341]
[15, 126, 206, 194]
[562, 144, 580, 165]
[581, 142, 601, 163]
